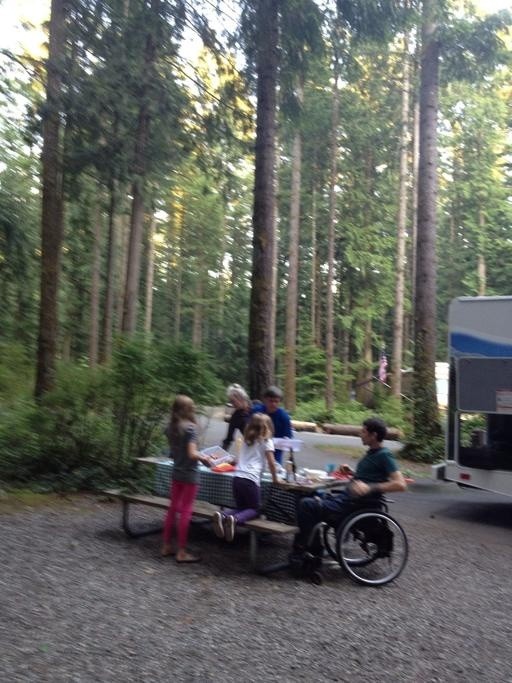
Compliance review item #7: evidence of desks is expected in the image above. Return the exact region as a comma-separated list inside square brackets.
[134, 454, 352, 572]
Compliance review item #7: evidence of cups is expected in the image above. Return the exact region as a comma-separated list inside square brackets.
[326, 462, 338, 472]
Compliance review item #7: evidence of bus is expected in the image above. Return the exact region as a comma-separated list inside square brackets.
[430, 294, 512, 500]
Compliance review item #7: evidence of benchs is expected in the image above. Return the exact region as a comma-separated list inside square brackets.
[104, 489, 299, 568]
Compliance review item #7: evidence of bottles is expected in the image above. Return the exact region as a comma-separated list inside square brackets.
[288, 447, 296, 480]
[286, 461, 294, 482]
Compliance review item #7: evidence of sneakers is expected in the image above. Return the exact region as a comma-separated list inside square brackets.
[162, 549, 201, 563]
[288, 545, 322, 565]
[213, 511, 236, 541]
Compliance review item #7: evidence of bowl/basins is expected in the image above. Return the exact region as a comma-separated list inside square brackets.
[305, 469, 327, 480]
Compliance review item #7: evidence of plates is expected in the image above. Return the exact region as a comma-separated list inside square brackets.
[319, 476, 336, 482]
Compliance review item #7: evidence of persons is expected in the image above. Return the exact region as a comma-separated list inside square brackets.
[160, 394, 215, 565]
[222, 381, 262, 452]
[249, 387, 298, 473]
[212, 411, 285, 540]
[288, 415, 410, 568]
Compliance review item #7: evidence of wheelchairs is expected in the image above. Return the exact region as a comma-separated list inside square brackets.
[288, 476, 415, 588]
